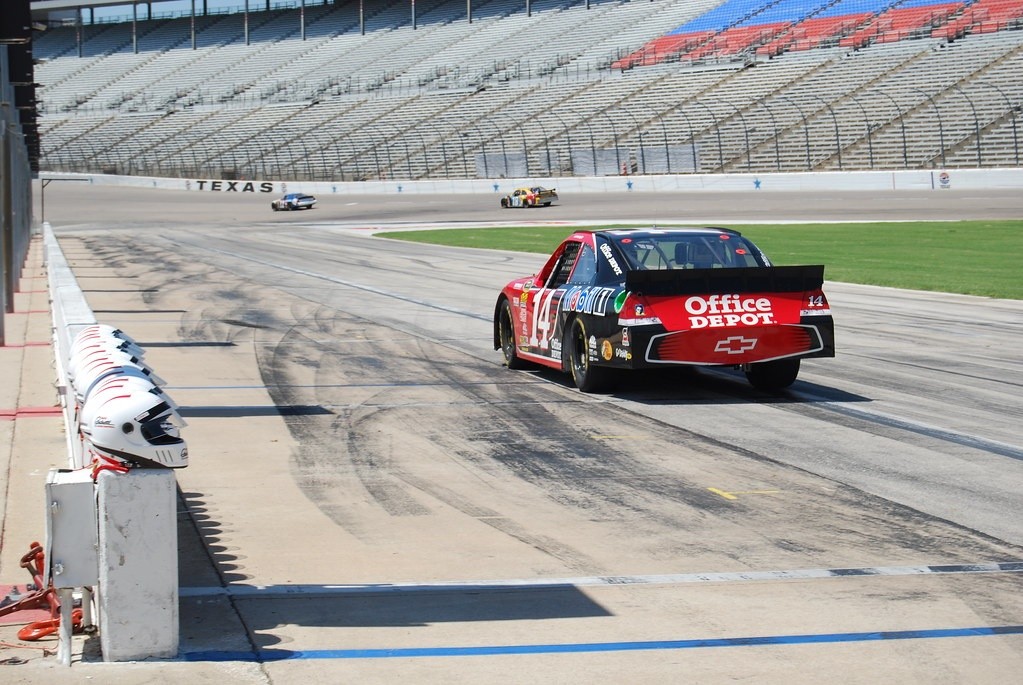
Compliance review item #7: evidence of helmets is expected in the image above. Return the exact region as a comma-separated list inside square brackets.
[70, 324, 189, 438]
[79, 376, 189, 468]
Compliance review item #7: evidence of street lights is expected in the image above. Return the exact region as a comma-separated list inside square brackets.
[622, 162, 628, 176]
[812, 96, 841, 169]
[838, 93, 874, 169]
[936, 85, 982, 167]
[42, 99, 752, 172]
[907, 87, 946, 168]
[786, 100, 811, 171]
[975, 81, 1018, 165]
[748, 97, 780, 172]
[870, 89, 908, 169]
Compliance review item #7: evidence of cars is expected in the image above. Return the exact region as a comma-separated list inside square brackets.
[501, 186, 559, 207]
[271, 192, 317, 212]
[492, 223, 835, 393]
